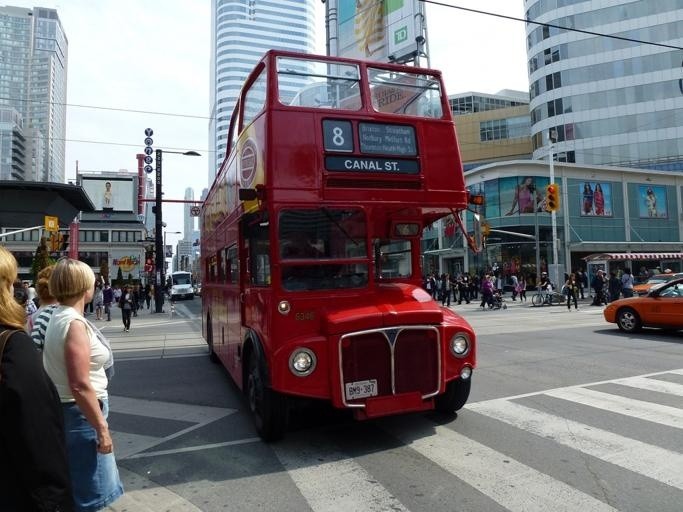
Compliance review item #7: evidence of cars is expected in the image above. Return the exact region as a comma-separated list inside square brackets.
[191, 281, 203, 296]
[603, 269, 683, 335]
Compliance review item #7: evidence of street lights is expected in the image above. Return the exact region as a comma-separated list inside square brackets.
[152, 147, 201, 314]
[162, 229, 181, 276]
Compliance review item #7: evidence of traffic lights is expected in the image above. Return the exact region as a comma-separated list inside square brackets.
[145, 251, 151, 265]
[58, 232, 69, 252]
[472, 195, 482, 205]
[548, 187, 556, 208]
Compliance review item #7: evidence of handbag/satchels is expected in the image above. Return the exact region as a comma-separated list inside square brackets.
[552, 282, 556, 290]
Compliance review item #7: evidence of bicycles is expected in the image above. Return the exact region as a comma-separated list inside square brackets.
[531, 283, 559, 306]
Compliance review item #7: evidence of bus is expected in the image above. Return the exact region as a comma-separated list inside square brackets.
[169, 269, 194, 300]
[194, 46, 477, 444]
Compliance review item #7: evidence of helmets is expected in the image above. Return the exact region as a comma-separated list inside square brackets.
[541, 272, 547, 275]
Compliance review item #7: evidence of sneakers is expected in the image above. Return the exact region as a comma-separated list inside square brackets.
[430, 295, 609, 312]
[84, 305, 150, 332]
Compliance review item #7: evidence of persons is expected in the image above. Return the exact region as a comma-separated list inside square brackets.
[84, 280, 151, 332]
[32, 263, 62, 353]
[0, 246, 73, 512]
[15, 280, 39, 316]
[593, 184, 604, 216]
[505, 176, 546, 215]
[423, 263, 672, 312]
[43, 256, 124, 512]
[646, 187, 657, 217]
[102, 182, 113, 211]
[583, 183, 593, 215]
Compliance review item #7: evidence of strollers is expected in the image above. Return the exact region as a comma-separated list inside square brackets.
[488, 290, 504, 310]
